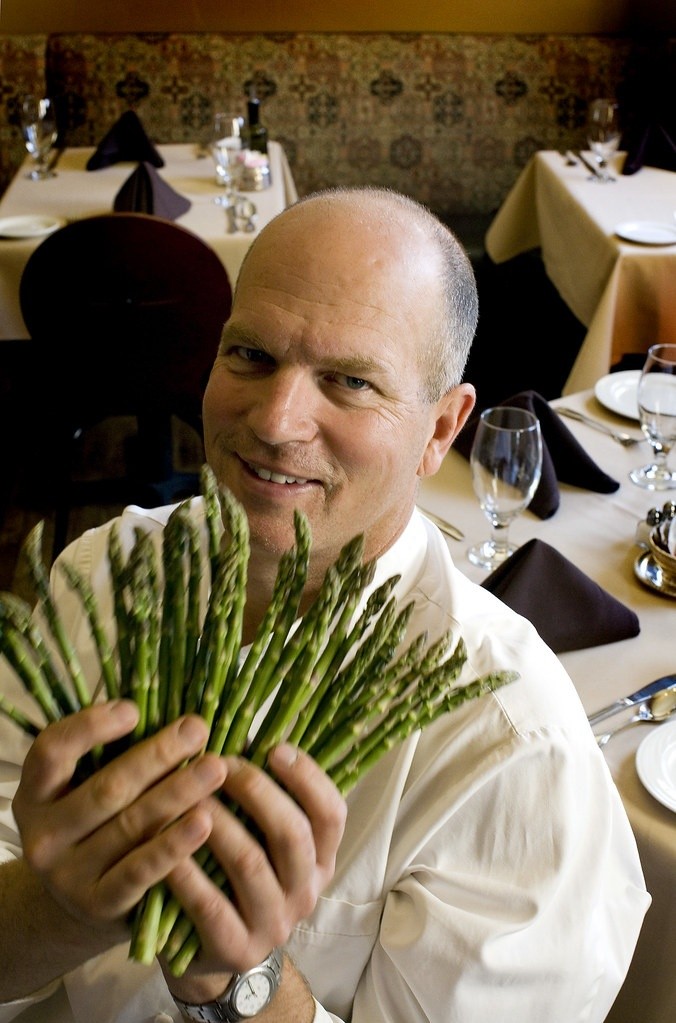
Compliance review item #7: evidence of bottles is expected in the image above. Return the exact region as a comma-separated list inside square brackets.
[243, 97, 269, 152]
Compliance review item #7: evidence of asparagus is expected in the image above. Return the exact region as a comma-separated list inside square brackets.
[0, 466, 522, 977]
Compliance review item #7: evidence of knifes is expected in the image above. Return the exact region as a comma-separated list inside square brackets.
[583, 672, 676, 726]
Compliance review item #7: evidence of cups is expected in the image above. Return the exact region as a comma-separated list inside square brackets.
[20, 94, 269, 233]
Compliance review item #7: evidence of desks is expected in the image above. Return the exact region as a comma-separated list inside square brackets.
[423, 363, 676, 1023]
[0, 140, 301, 339]
[485, 147, 676, 395]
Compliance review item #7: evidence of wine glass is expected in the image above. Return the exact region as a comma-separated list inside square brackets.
[587, 100, 620, 186]
[466, 407, 542, 571]
[630, 344, 676, 491]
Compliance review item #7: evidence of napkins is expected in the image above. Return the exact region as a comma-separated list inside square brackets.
[482, 537, 641, 671]
[87, 111, 166, 171]
[454, 392, 621, 523]
[624, 118, 676, 176]
[113, 163, 190, 221]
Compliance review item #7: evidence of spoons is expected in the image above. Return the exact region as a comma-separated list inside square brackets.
[594, 688, 676, 747]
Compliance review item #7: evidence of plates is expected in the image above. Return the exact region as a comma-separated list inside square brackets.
[633, 550, 676, 599]
[595, 369, 638, 422]
[635, 721, 676, 814]
[615, 219, 676, 245]
[1, 217, 63, 238]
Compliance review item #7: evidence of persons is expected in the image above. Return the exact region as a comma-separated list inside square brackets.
[0, 186, 651, 1022]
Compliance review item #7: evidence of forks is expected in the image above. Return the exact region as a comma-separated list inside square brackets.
[554, 408, 647, 447]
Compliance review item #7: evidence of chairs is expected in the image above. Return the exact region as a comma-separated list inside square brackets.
[16, 215, 238, 573]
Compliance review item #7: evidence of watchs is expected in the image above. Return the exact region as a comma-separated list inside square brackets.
[170, 944, 284, 1023]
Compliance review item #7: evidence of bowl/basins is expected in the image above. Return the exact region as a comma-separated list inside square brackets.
[648, 530, 676, 590]
